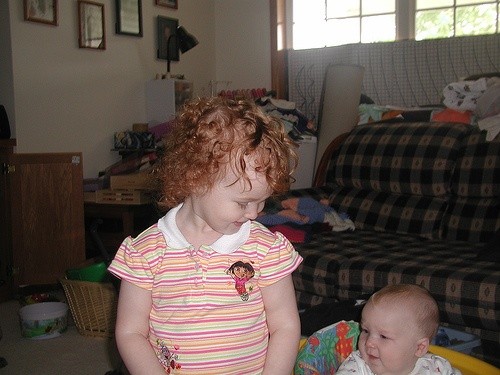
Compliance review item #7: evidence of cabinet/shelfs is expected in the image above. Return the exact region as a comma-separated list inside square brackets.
[146, 78, 192, 126]
[1, 152, 83, 288]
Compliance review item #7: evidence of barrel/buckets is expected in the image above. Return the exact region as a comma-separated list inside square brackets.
[18, 302, 68, 340]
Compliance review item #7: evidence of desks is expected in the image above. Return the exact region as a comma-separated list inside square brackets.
[83, 201, 159, 287]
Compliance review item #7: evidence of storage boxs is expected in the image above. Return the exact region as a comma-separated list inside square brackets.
[94, 131, 162, 206]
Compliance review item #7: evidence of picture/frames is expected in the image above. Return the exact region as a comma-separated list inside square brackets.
[115, 0, 143, 36]
[155, 0, 178, 9]
[23, 0, 60, 26]
[77, 0, 106, 50]
[157, 15, 180, 62]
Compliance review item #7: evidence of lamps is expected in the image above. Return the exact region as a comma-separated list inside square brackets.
[167, 25, 200, 73]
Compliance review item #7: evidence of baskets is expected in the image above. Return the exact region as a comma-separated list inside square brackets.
[57, 276, 118, 338]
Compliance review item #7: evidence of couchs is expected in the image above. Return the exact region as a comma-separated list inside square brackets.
[262, 119, 500, 371]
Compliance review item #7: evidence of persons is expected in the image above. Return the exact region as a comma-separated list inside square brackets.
[333, 283, 460, 374]
[108, 95, 305, 374]
